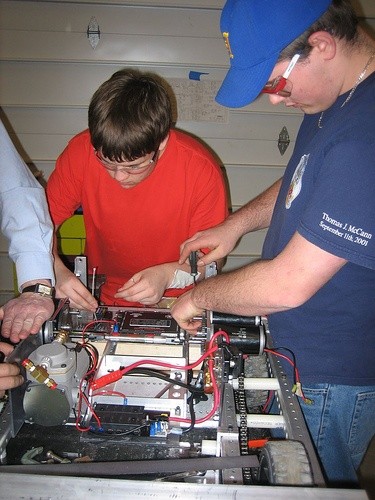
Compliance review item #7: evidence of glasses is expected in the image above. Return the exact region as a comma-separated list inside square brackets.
[261, 45, 304, 97]
[94, 147, 159, 175]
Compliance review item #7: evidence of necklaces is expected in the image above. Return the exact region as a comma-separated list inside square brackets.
[318, 52, 375, 128]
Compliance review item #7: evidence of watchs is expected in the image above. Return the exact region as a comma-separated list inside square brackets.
[22, 283, 56, 299]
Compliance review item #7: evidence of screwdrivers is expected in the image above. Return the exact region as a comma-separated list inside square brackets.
[189, 248, 203, 286]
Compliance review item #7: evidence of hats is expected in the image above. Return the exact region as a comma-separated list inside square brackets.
[214, 0, 333, 108]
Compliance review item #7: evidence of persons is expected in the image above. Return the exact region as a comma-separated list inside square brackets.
[0, 118, 56, 398]
[170, 0, 375, 489]
[45, 69, 229, 313]
